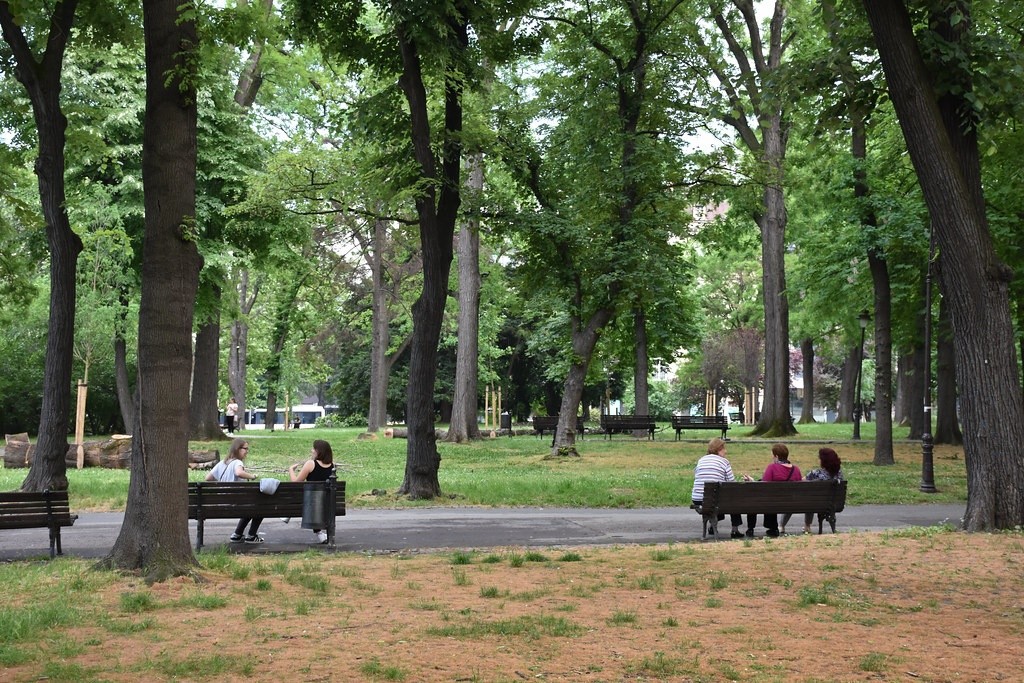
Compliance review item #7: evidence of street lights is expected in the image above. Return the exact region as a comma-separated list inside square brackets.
[850, 309, 872, 440]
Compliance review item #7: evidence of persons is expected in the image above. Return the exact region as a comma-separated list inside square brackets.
[293, 414, 301, 429]
[741, 444, 802, 537]
[778, 448, 845, 532]
[691, 437, 745, 538]
[218, 406, 224, 412]
[206, 438, 264, 544]
[279, 440, 335, 543]
[225, 396, 238, 436]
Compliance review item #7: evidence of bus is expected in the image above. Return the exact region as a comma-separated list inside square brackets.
[219, 404, 326, 432]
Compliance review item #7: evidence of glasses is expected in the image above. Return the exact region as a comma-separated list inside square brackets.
[241, 447, 247, 449]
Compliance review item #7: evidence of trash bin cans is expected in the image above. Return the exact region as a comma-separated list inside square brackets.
[300, 484, 328, 530]
[501, 414, 510, 428]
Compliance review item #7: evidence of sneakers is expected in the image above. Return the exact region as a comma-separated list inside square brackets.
[245, 534, 265, 544]
[230, 532, 244, 544]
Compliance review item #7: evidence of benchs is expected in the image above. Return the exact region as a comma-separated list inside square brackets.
[730, 412, 745, 423]
[691, 481, 846, 540]
[599, 414, 660, 439]
[671, 414, 732, 441]
[754, 413, 795, 424]
[220, 418, 242, 433]
[0, 492, 79, 559]
[532, 416, 590, 442]
[185, 479, 348, 555]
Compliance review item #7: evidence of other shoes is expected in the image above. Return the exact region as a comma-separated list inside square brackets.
[731, 530, 744, 538]
[318, 532, 328, 542]
[802, 527, 810, 532]
[746, 528, 754, 536]
[779, 526, 785, 533]
[708, 527, 718, 534]
[766, 529, 779, 536]
